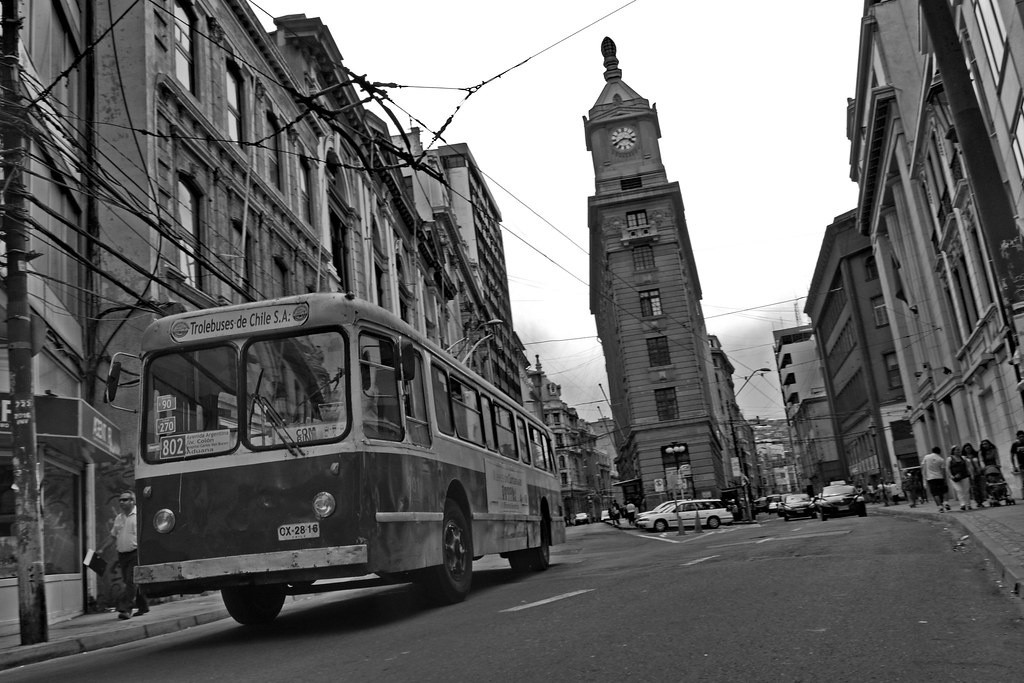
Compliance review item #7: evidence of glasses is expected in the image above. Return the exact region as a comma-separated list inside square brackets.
[118, 498, 132, 502]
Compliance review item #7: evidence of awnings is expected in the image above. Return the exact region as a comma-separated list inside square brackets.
[611, 478, 641, 486]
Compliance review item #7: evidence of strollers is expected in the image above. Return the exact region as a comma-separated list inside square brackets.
[982, 464, 1017, 506]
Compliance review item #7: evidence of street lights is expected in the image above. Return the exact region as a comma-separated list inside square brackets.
[869, 421, 891, 508]
[666, 443, 688, 499]
[726, 366, 771, 470]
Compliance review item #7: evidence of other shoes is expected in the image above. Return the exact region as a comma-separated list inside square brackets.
[939, 509, 944, 512]
[133, 605, 149, 616]
[960, 505, 965, 510]
[945, 504, 950, 510]
[119, 609, 132, 621]
[978, 504, 985, 507]
[968, 506, 972, 510]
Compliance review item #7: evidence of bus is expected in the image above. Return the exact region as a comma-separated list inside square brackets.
[102, 292, 566, 626]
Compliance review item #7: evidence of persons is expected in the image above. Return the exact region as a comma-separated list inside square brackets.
[902, 469, 915, 507]
[727, 498, 748, 522]
[857, 481, 899, 506]
[608, 499, 636, 527]
[95, 489, 150, 620]
[1010, 430, 1024, 500]
[921, 440, 1000, 513]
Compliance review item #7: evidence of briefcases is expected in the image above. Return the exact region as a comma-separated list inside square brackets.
[83, 549, 108, 577]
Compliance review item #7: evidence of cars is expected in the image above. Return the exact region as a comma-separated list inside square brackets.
[600, 509, 609, 521]
[775, 493, 791, 514]
[633, 497, 735, 533]
[573, 512, 589, 524]
[814, 484, 867, 521]
[781, 493, 818, 521]
[767, 493, 779, 515]
